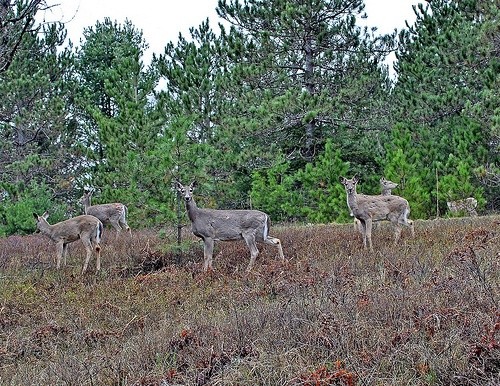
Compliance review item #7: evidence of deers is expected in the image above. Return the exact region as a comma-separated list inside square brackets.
[175, 180, 285, 277]
[339, 173, 415, 253]
[446, 192, 478, 218]
[78, 189, 133, 241]
[32, 209, 104, 275]
